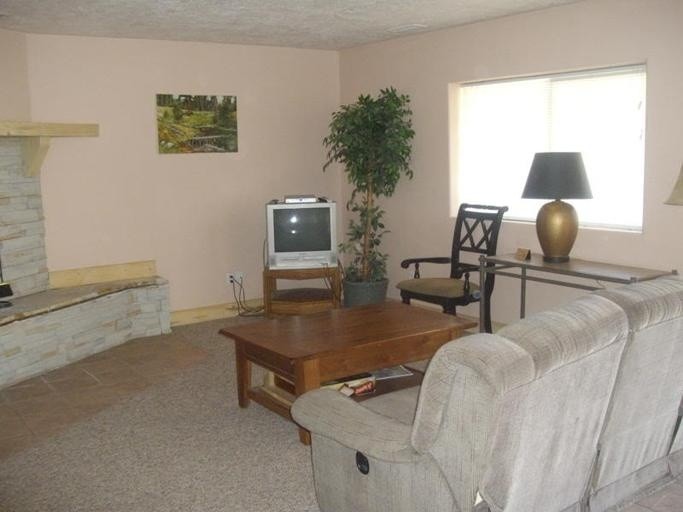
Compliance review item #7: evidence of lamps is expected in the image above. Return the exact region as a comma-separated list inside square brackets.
[522, 153, 593, 262]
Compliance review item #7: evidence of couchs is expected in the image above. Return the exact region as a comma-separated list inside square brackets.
[290, 274, 682, 512]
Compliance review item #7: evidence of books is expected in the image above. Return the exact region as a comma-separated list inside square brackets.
[320, 373, 375, 397]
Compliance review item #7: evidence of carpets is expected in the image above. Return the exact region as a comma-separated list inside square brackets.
[0, 318, 682, 512]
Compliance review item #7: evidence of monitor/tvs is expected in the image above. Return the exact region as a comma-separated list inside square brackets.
[265, 201, 340, 272]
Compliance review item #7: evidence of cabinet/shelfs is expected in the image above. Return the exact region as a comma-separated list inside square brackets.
[260, 269, 339, 315]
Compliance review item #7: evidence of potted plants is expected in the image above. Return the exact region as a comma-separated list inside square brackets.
[320, 86, 416, 308]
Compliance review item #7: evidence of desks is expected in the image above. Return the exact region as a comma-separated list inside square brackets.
[478, 255, 678, 330]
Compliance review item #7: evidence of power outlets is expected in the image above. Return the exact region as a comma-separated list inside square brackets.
[226, 272, 235, 285]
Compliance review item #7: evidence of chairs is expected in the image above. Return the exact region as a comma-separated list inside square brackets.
[396, 203, 507, 313]
